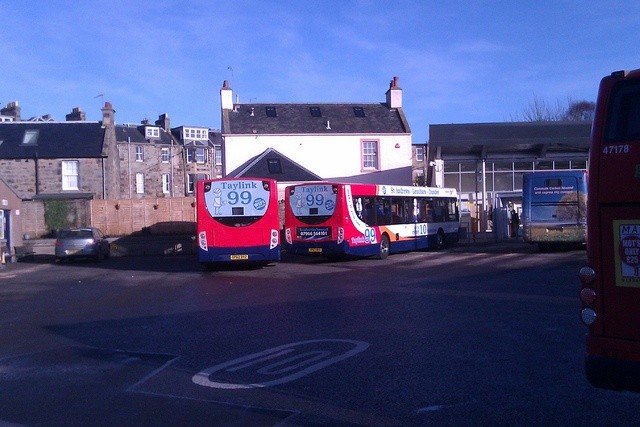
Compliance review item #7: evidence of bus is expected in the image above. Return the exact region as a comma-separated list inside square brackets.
[522, 170, 588, 246]
[198, 178, 281, 269]
[580, 67, 640, 395]
[285, 181, 461, 260]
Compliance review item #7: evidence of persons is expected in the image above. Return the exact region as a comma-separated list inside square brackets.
[509, 209, 520, 241]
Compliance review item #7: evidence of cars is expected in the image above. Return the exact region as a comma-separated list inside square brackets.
[55, 227, 110, 262]
